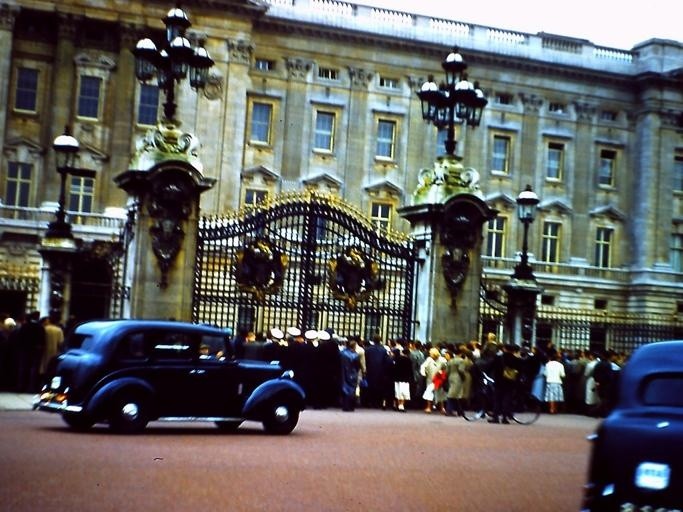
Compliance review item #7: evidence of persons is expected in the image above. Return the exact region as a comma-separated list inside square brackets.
[1, 308, 79, 394]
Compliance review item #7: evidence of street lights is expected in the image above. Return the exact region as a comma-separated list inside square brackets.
[414, 44, 486, 157]
[43, 124, 80, 239]
[510, 184, 539, 280]
[130, 6, 215, 121]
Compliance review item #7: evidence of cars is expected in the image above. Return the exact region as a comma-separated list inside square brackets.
[582, 339, 682, 510]
[32, 320, 306, 435]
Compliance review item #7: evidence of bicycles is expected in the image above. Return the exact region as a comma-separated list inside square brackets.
[460, 370, 540, 424]
